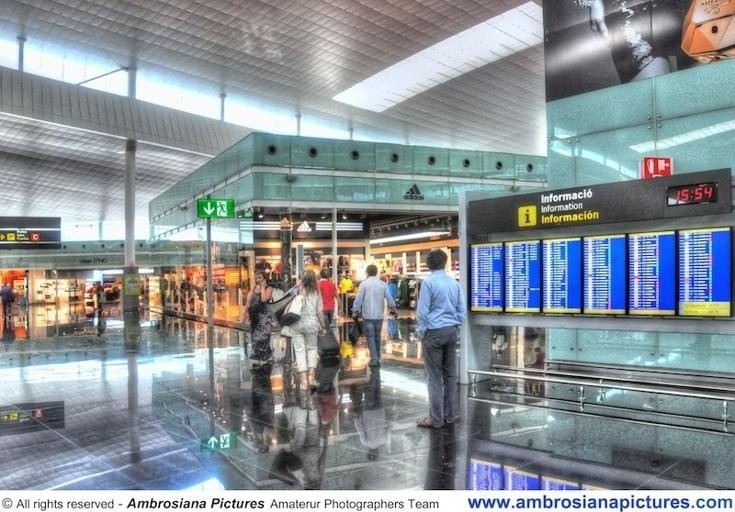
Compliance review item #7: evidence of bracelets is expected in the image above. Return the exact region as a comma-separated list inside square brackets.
[322, 326, 325, 330]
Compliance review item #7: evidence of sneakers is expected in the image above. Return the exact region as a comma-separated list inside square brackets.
[299, 376, 321, 390]
[415, 415, 458, 428]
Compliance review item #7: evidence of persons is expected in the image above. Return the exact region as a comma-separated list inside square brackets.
[242, 270, 273, 374]
[338, 274, 352, 317]
[306, 255, 320, 273]
[159, 273, 205, 310]
[415, 248, 464, 429]
[248, 369, 342, 489]
[95, 282, 104, 309]
[340, 338, 352, 370]
[97, 310, 107, 336]
[283, 270, 327, 390]
[352, 265, 396, 367]
[18, 285, 24, 301]
[350, 365, 387, 460]
[320, 268, 342, 332]
[3, 317, 15, 352]
[140, 285, 149, 295]
[384, 273, 397, 315]
[0, 282, 12, 319]
[88, 283, 95, 299]
[387, 317, 396, 341]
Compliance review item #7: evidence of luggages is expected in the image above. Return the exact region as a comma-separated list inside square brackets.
[314, 354, 342, 385]
[316, 327, 341, 355]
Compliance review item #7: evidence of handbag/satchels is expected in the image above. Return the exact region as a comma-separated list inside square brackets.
[279, 293, 304, 327]
[348, 319, 363, 344]
